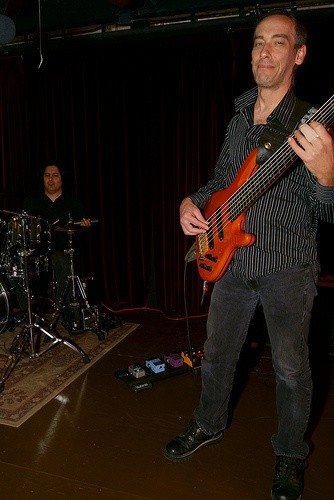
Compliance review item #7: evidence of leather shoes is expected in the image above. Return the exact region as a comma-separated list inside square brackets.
[270, 456, 308, 500]
[164, 418, 223, 462]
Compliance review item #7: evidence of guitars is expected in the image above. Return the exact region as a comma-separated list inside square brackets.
[194, 94, 334, 282]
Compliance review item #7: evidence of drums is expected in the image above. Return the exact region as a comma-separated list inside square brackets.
[0, 281, 14, 335]
[0, 250, 27, 281]
[7, 214, 44, 259]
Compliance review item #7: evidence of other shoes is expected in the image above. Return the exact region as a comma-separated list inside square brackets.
[62, 304, 74, 323]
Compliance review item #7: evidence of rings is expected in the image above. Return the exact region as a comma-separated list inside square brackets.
[308, 135, 319, 142]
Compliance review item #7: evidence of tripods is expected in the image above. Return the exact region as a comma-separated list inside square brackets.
[0, 209, 119, 392]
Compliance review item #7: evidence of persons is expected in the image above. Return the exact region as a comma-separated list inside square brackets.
[13, 159, 91, 312]
[163, 11, 334, 500]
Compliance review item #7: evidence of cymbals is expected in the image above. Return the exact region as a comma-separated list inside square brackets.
[54, 225, 81, 233]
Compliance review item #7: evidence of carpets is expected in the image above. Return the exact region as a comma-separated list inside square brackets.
[0, 317, 141, 428]
